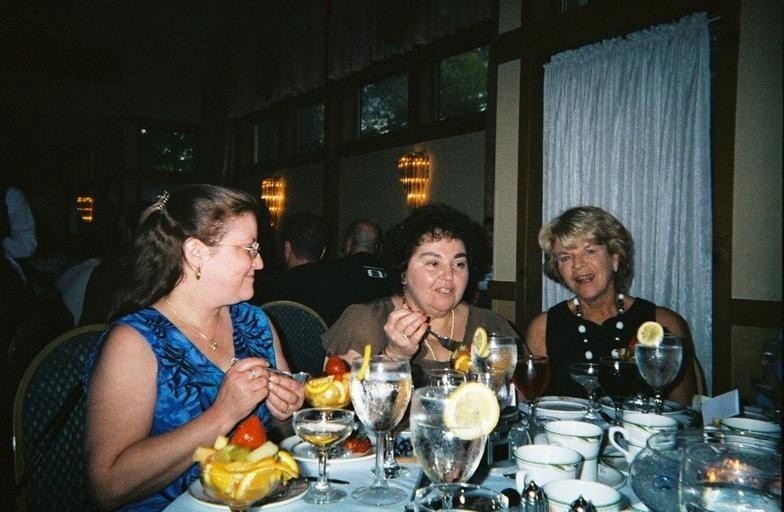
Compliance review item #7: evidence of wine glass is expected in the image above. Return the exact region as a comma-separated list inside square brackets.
[292, 332, 519, 511]
[511, 331, 685, 430]
[196, 463, 282, 512]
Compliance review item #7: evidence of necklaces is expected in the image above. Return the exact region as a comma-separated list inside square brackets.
[572, 294, 626, 376]
[423, 307, 459, 362]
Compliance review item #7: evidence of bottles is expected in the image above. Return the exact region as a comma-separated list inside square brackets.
[751, 339, 782, 418]
[522, 480, 549, 511]
[569, 495, 596, 511]
[500, 487, 522, 510]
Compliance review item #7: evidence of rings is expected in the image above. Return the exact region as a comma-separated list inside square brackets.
[281, 403, 293, 417]
[246, 369, 256, 379]
[402, 332, 410, 341]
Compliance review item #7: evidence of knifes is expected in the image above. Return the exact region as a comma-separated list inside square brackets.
[298, 476, 347, 484]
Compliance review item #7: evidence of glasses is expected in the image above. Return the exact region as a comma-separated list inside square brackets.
[212, 240, 260, 260]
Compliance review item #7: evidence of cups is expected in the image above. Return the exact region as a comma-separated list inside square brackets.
[702, 417, 780, 455]
[514, 419, 622, 512]
[608, 413, 678, 464]
[675, 441, 782, 511]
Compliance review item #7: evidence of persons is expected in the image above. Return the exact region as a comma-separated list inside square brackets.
[519, 205, 700, 407]
[78, 184, 306, 510]
[317, 200, 532, 387]
[2, 176, 401, 362]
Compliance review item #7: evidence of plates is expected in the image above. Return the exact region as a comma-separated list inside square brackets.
[186, 460, 311, 507]
[279, 434, 377, 461]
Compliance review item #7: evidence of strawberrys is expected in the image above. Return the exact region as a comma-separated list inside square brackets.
[343, 431, 373, 453]
[229, 415, 267, 452]
[325, 354, 347, 375]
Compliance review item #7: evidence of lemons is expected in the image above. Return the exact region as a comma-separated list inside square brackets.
[443, 382, 501, 441]
[637, 321, 665, 347]
[357, 344, 371, 381]
[306, 375, 352, 406]
[471, 327, 488, 357]
[194, 436, 299, 504]
